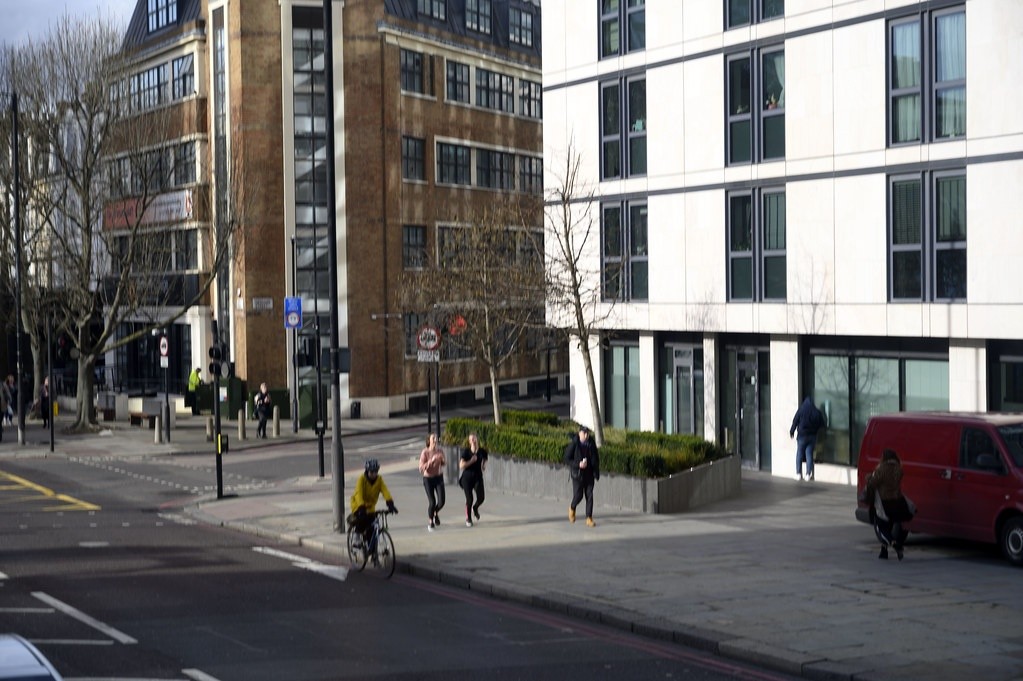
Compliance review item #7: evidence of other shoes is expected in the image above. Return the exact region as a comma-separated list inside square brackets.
[893, 542, 904, 560]
[879, 546, 889, 559]
[568, 506, 576, 524]
[794, 473, 802, 481]
[434, 511, 440, 526]
[465, 519, 473, 528]
[352, 533, 361, 546]
[370, 555, 382, 568]
[586, 517, 595, 527]
[256, 433, 268, 440]
[473, 506, 481, 520]
[429, 517, 435, 528]
[804, 474, 811, 482]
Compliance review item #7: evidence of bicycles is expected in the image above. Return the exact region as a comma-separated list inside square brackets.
[347, 509, 396, 578]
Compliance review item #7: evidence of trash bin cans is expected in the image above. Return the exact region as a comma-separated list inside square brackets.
[299, 384, 327, 431]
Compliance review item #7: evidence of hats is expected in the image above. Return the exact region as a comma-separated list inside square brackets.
[579, 427, 591, 433]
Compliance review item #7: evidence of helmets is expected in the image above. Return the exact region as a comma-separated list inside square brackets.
[365, 458, 381, 472]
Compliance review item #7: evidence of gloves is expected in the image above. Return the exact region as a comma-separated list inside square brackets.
[385, 499, 399, 514]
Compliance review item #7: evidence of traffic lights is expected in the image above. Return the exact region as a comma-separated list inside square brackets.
[209, 342, 224, 377]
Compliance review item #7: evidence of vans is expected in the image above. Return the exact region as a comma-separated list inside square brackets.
[854, 411, 1023, 569]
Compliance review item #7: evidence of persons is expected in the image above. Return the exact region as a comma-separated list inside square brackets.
[459, 432, 488, 528]
[419, 431, 446, 527]
[254, 382, 271, 438]
[790, 397, 825, 481]
[40, 375, 57, 429]
[188, 368, 203, 415]
[565, 427, 600, 527]
[351, 458, 398, 568]
[863, 449, 916, 561]
[0, 375, 17, 444]
